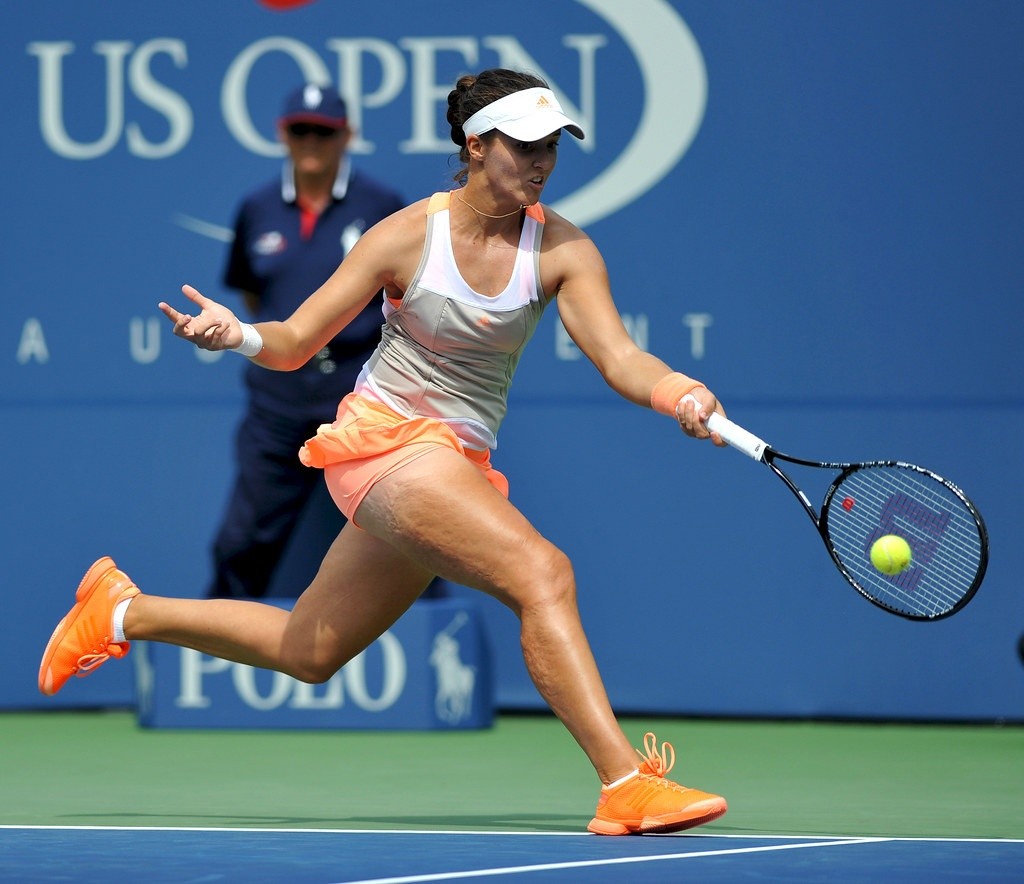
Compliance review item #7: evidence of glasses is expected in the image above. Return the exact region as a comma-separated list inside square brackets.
[283, 123, 337, 140]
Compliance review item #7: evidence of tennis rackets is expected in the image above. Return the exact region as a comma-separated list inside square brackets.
[674, 394, 989, 622]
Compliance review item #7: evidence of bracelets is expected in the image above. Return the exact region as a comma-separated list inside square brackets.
[229, 317, 263, 356]
[651, 372, 707, 418]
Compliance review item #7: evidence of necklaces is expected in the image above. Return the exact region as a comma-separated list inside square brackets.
[454, 191, 531, 218]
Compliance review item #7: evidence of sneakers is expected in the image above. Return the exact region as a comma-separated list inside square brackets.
[38, 556, 142, 695]
[587, 733, 725, 836]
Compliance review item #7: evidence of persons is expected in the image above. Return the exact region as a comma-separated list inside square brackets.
[205, 83, 408, 601]
[38, 69, 728, 835]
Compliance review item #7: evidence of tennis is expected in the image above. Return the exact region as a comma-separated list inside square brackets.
[870, 534, 913, 577]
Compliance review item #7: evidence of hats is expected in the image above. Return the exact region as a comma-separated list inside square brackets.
[279, 84, 347, 129]
[462, 88, 585, 143]
